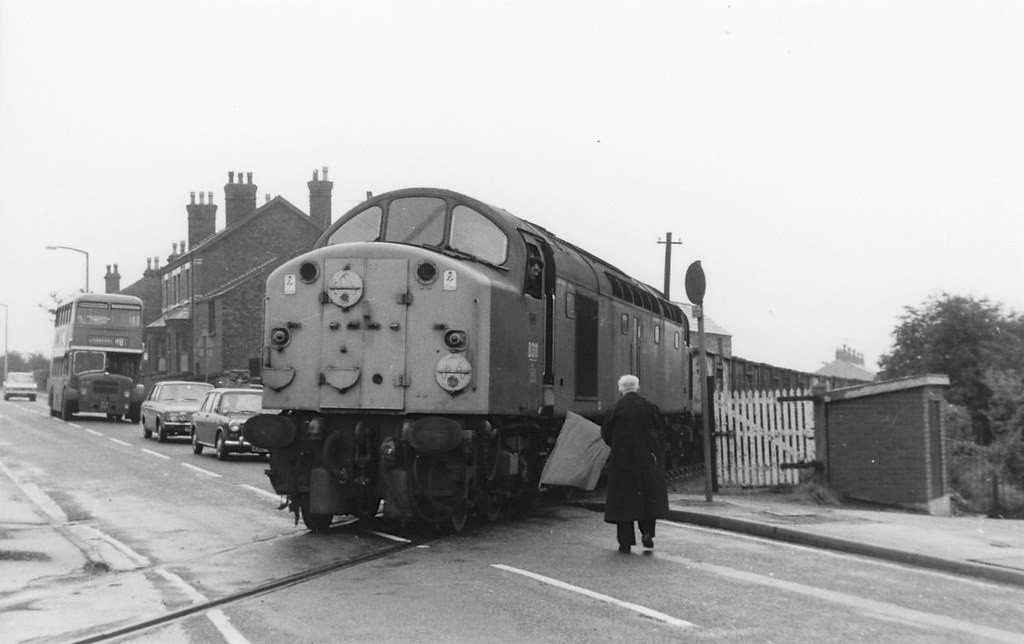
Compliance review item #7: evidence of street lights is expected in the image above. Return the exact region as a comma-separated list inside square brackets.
[46, 246, 89, 293]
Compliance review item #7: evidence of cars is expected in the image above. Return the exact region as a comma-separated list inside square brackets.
[140, 382, 215, 443]
[3, 372, 38, 402]
[190, 388, 283, 462]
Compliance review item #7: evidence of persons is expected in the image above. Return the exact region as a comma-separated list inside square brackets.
[524, 257, 544, 299]
[601, 375, 670, 555]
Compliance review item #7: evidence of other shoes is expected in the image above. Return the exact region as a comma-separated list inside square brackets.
[619, 543, 631, 553]
[642, 534, 653, 548]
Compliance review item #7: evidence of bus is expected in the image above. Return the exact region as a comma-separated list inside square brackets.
[46, 293, 145, 424]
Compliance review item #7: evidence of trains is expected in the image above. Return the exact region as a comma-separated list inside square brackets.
[242, 187, 874, 537]
